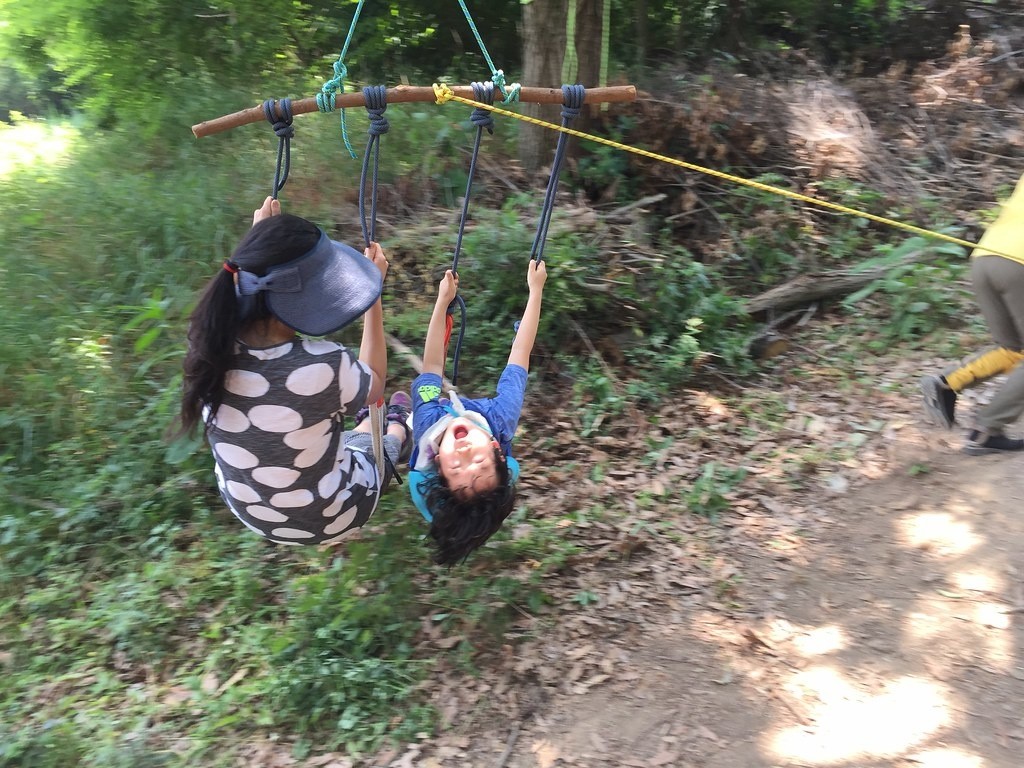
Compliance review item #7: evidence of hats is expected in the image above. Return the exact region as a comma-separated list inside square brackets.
[237, 222, 381, 337]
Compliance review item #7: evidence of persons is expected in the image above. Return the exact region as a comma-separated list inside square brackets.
[162, 196, 414, 545]
[918, 167, 1024, 454]
[402, 257, 548, 564]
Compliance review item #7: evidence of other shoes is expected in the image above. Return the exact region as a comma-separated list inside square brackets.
[924, 374, 957, 431]
[963, 429, 1024, 457]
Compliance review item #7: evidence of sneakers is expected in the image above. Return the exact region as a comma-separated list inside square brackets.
[355, 406, 370, 426]
[384, 390, 414, 464]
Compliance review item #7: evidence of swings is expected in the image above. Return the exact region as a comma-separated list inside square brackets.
[187, 1, 639, 549]
